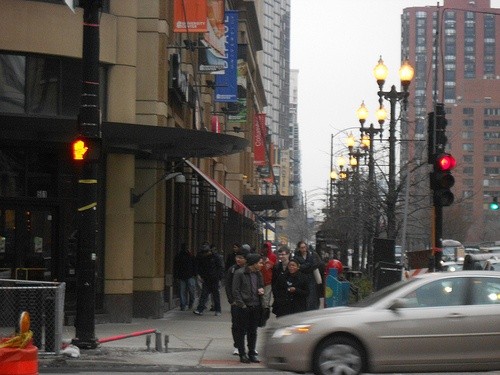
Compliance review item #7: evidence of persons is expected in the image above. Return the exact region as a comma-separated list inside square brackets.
[173, 240, 324, 363]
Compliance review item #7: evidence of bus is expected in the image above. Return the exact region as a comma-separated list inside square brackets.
[441, 238, 465, 261]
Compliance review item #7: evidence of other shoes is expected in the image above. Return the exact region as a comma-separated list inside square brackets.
[233, 348, 239, 355]
[248, 354, 260, 363]
[240, 352, 249, 364]
[179, 306, 222, 316]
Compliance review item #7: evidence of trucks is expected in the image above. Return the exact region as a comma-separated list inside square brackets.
[394, 245, 408, 268]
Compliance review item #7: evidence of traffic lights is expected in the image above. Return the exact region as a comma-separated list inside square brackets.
[68, 134, 101, 170]
[426, 108, 448, 165]
[430, 151, 457, 208]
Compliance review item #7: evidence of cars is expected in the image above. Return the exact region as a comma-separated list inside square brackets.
[442, 261, 464, 273]
[259, 268, 500, 375]
[482, 254, 500, 270]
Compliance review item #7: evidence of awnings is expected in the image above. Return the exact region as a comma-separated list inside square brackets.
[182, 158, 277, 232]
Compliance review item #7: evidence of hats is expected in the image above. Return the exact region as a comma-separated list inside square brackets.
[246, 254, 261, 265]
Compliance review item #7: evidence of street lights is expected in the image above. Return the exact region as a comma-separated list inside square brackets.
[337, 154, 358, 189]
[373, 52, 415, 239]
[345, 130, 371, 272]
[330, 168, 347, 197]
[354, 99, 386, 188]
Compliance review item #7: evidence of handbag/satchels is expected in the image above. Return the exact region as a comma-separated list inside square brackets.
[258, 308, 267, 327]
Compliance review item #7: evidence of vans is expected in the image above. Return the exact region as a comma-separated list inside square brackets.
[462, 251, 500, 270]
